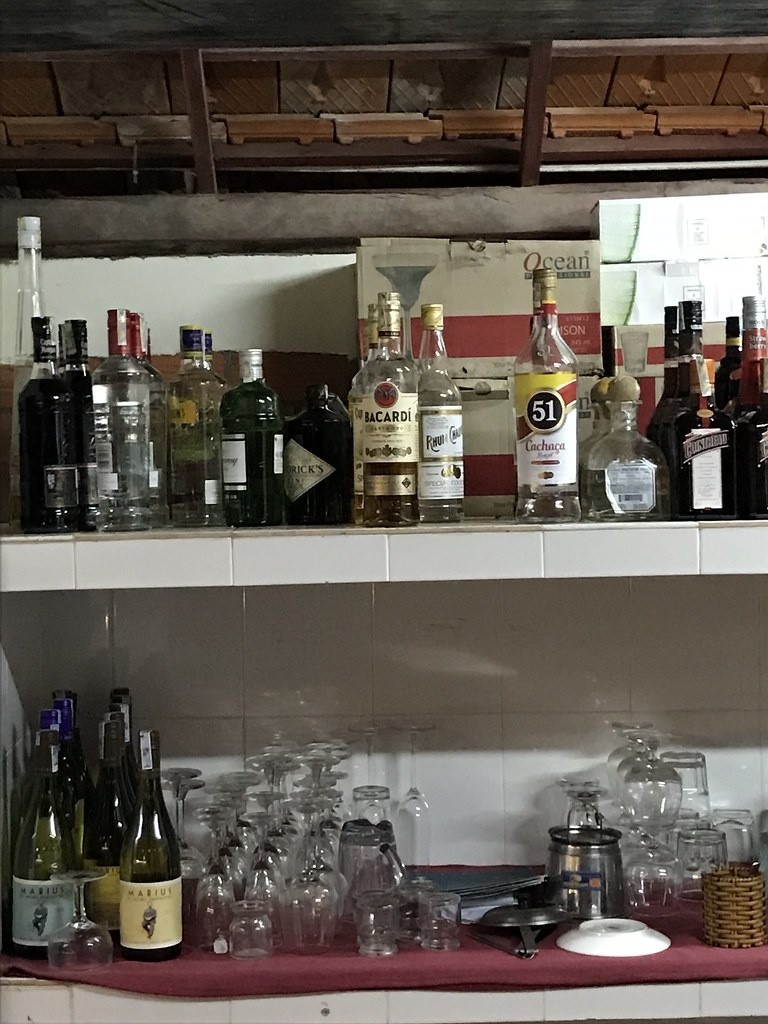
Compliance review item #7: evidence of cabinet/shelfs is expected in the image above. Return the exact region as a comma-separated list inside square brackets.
[0, 518, 768, 1023]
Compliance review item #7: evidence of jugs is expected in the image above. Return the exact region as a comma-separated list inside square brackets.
[340, 820, 408, 925]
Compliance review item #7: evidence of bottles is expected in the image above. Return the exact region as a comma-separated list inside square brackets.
[350, 293, 465, 526]
[13, 687, 181, 962]
[514, 267, 768, 522]
[11, 216, 287, 535]
[289, 382, 351, 527]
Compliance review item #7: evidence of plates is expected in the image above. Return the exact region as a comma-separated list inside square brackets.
[557, 919, 671, 957]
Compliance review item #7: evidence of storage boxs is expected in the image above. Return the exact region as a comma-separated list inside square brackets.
[357, 192, 767, 516]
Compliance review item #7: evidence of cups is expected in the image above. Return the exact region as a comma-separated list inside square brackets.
[355, 890, 398, 956]
[353, 787, 391, 821]
[228, 904, 274, 960]
[418, 894, 461, 949]
[562, 775, 605, 827]
[658, 755, 761, 905]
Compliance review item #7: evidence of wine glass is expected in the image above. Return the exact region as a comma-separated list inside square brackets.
[348, 723, 391, 786]
[51, 870, 114, 972]
[395, 722, 434, 868]
[163, 753, 350, 951]
[609, 720, 682, 916]
